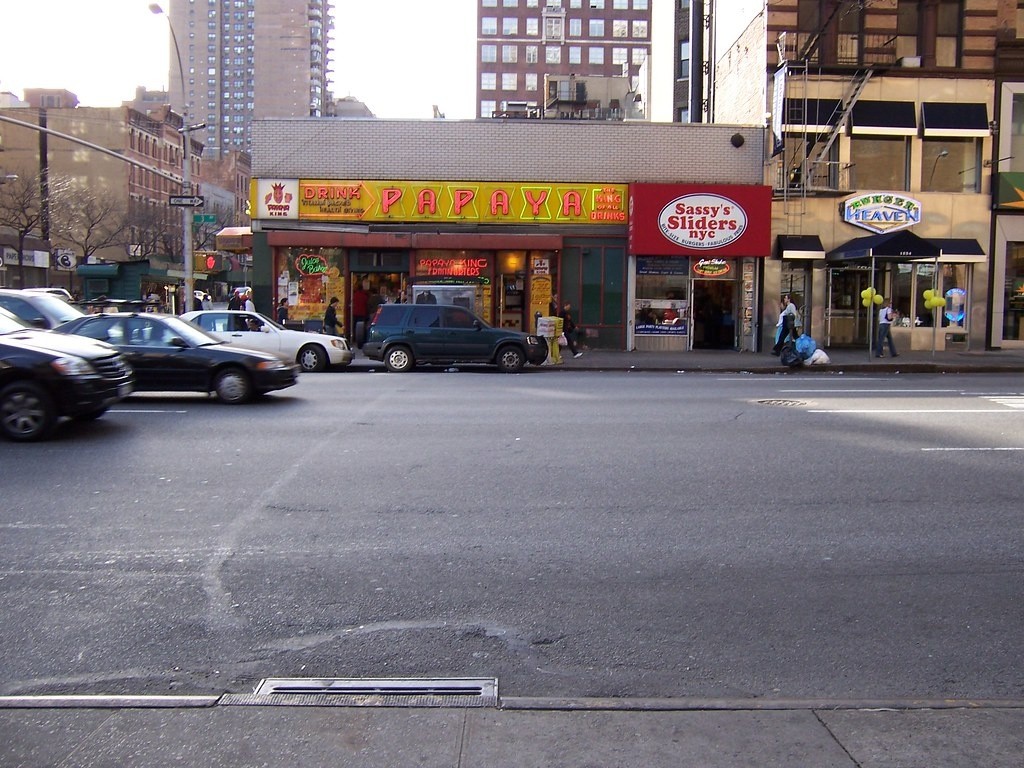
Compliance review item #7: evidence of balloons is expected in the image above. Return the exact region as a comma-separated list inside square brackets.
[923, 287, 946, 308]
[861, 287, 884, 306]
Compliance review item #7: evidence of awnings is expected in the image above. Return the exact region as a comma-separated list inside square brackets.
[777, 234, 825, 258]
[911, 239, 987, 262]
[78, 265, 120, 276]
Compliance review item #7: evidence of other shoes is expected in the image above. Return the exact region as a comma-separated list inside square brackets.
[892, 353, 900, 357]
[771, 351, 780, 357]
[876, 354, 885, 357]
[574, 353, 583, 358]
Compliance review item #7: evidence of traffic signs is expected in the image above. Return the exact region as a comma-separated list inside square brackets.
[169, 195, 204, 207]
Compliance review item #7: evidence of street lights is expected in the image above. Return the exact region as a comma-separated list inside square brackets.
[148, 2, 195, 314]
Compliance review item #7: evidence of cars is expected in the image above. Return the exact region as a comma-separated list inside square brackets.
[0, 306, 133, 442]
[193, 289, 211, 303]
[0, 288, 85, 330]
[52, 312, 302, 404]
[228, 287, 251, 301]
[120, 310, 355, 372]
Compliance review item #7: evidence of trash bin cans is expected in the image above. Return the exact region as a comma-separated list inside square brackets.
[286, 319, 305, 331]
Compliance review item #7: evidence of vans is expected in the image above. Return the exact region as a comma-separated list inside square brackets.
[23, 288, 74, 301]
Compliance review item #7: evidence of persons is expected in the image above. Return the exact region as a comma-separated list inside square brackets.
[183, 292, 214, 311]
[402, 290, 408, 304]
[702, 295, 722, 348]
[324, 297, 343, 336]
[277, 298, 291, 330]
[353, 287, 368, 322]
[771, 295, 803, 356]
[227, 290, 255, 327]
[549, 292, 558, 316]
[875, 298, 900, 358]
[248, 320, 261, 332]
[87, 292, 165, 313]
[416, 290, 437, 304]
[368, 293, 383, 320]
[536, 259, 546, 273]
[558, 303, 583, 359]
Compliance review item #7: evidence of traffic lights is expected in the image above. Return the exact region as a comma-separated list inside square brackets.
[205, 255, 223, 271]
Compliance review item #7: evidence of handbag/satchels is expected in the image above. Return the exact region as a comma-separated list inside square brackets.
[885, 309, 894, 321]
[558, 332, 567, 346]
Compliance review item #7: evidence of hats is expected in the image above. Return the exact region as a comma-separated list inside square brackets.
[235, 290, 239, 294]
[330, 297, 338, 305]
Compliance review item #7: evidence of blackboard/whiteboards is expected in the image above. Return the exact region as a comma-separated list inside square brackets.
[304, 319, 324, 334]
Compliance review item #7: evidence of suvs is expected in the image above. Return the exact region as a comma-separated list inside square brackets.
[354, 304, 550, 373]
